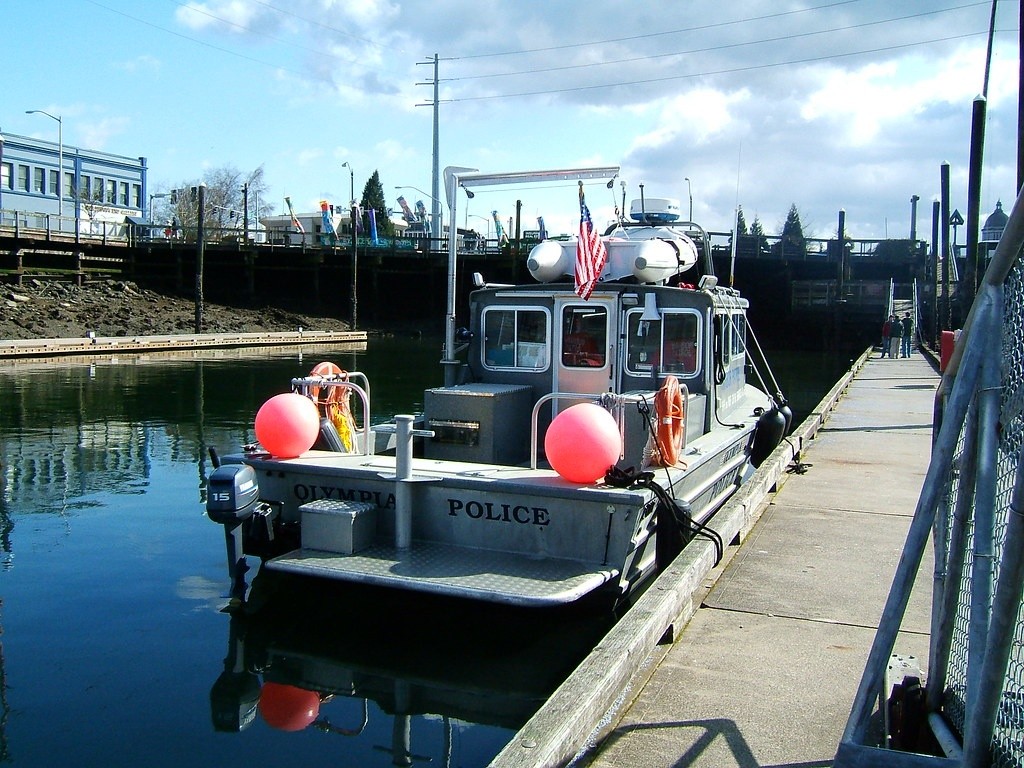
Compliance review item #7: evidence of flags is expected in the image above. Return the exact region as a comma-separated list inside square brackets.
[574, 192, 608, 301]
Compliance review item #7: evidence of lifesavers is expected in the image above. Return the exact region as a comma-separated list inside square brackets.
[655, 375, 685, 467]
[308, 361, 343, 418]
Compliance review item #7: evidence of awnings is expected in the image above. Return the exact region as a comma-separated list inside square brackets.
[126, 216, 150, 225]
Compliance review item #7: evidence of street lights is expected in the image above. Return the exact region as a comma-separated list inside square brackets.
[468, 215, 489, 247]
[240, 185, 258, 234]
[342, 162, 353, 199]
[26, 110, 62, 233]
[394, 186, 443, 253]
[685, 177, 692, 230]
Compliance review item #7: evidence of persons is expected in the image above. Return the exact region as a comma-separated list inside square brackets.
[164, 217, 179, 240]
[881, 311, 914, 359]
[463, 229, 487, 254]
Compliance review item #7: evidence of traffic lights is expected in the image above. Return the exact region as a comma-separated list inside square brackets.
[230, 211, 234, 218]
[213, 208, 216, 214]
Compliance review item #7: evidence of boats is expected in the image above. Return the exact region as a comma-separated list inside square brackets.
[204, 166, 793, 612]
[208, 573, 621, 768]
